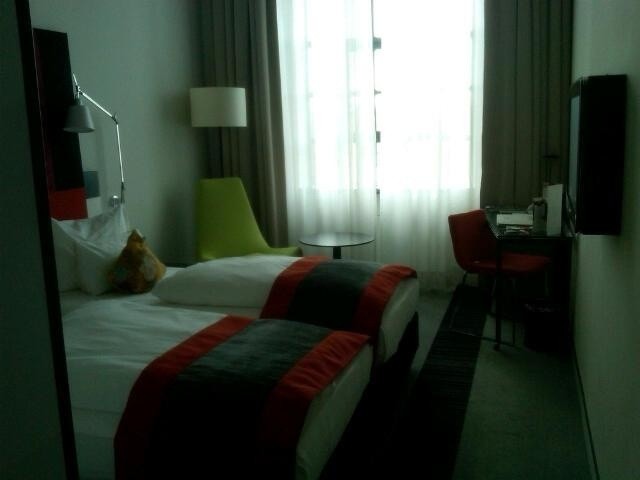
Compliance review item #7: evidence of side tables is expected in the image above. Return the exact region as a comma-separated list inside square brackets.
[298, 232, 374, 259]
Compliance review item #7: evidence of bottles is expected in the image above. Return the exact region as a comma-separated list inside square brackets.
[531, 198, 547, 235]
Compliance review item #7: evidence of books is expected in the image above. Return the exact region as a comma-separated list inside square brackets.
[495, 212, 534, 236]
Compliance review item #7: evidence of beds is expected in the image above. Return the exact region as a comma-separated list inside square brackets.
[59, 301, 374, 480]
[58, 256, 419, 384]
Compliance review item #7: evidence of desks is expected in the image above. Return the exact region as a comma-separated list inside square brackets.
[484, 208, 572, 351]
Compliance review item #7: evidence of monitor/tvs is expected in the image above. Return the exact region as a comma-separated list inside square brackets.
[564, 74, 628, 236]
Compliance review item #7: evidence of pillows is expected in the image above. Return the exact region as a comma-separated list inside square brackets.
[106, 229, 167, 294]
[50, 218, 81, 292]
[74, 211, 129, 298]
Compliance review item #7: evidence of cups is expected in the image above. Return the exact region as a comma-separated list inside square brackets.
[543, 183, 563, 237]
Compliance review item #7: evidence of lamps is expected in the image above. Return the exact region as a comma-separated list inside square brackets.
[65, 72, 125, 208]
[187, 87, 247, 178]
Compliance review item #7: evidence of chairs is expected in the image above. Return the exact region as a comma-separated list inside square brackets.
[195, 176, 301, 263]
[449, 210, 550, 346]
